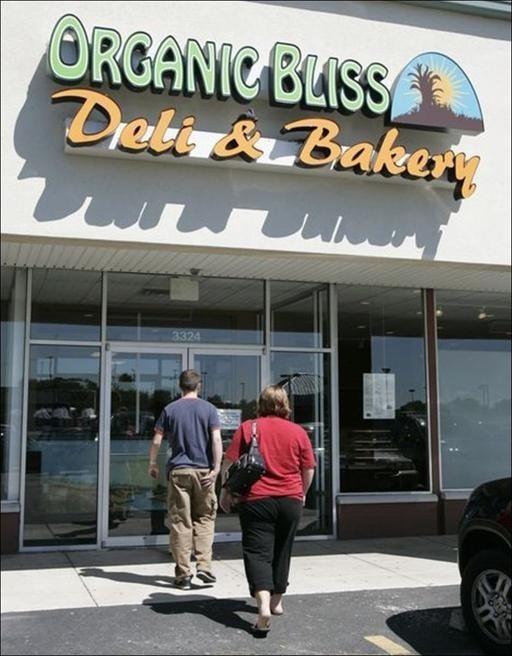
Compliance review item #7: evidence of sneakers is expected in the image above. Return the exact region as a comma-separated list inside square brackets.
[196, 570, 216, 583]
[173, 578, 192, 590]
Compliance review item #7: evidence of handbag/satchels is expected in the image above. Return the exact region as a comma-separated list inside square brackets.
[221, 447, 266, 498]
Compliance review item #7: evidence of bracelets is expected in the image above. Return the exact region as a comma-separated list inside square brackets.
[149, 461, 157, 465]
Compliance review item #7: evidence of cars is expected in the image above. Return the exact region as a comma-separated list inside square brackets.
[402, 407, 510, 480]
[32, 404, 235, 508]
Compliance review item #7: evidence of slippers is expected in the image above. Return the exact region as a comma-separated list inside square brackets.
[251, 622, 270, 632]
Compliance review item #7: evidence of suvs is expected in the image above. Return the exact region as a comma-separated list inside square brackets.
[302, 422, 417, 495]
[455, 472, 512, 654]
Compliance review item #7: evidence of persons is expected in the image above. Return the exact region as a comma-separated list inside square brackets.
[148, 369, 224, 590]
[218, 384, 317, 632]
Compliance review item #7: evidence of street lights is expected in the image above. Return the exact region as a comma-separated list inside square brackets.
[44, 354, 54, 382]
[128, 363, 247, 411]
[380, 368, 391, 404]
[407, 388, 417, 401]
